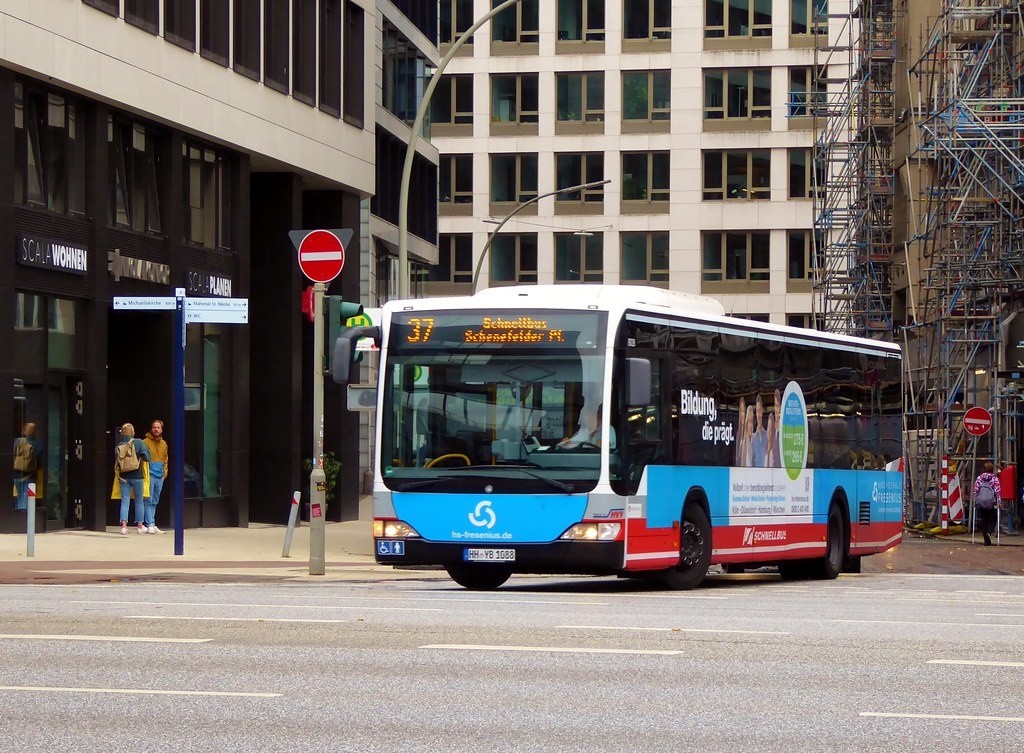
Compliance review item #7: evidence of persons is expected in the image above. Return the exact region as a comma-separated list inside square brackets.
[115, 423, 150, 534]
[561, 399, 616, 448]
[736, 389, 781, 467]
[14, 423, 42, 509]
[972, 461, 1002, 546]
[143, 420, 168, 534]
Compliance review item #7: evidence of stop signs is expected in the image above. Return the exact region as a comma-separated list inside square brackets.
[297, 231, 345, 282]
[962, 406, 992, 437]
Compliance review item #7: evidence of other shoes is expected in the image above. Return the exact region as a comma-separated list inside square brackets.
[985, 533, 992, 546]
[121, 528, 127, 535]
[155, 526, 164, 535]
[137, 525, 147, 534]
[148, 526, 154, 534]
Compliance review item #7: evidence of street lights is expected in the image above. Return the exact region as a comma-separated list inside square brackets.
[470, 180, 613, 297]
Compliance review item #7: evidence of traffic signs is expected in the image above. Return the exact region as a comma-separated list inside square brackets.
[186, 297, 248, 310]
[113, 297, 177, 309]
[185, 311, 248, 324]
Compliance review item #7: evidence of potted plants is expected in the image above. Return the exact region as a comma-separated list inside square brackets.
[301, 450, 343, 519]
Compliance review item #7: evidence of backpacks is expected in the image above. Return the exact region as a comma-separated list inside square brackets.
[14, 438, 36, 472]
[114, 438, 142, 473]
[976, 474, 997, 509]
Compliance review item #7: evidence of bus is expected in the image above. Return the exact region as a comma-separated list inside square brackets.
[333, 285, 906, 590]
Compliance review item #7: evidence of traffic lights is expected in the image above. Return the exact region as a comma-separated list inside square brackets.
[327, 294, 365, 377]
